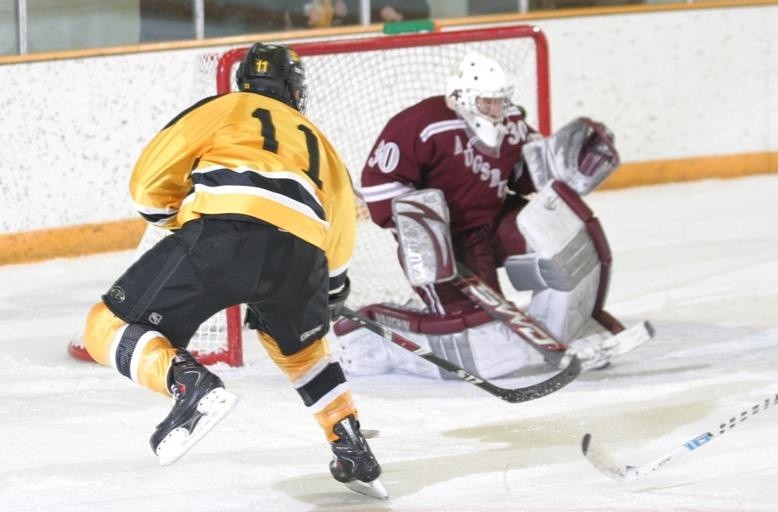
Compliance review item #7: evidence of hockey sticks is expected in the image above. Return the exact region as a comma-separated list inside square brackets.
[449, 262, 655, 373]
[581, 394, 777, 485]
[341, 307, 581, 403]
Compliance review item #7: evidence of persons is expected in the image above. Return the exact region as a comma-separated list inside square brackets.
[356, 51, 619, 317]
[85, 40, 396, 501]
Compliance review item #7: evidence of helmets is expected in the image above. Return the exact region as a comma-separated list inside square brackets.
[235, 42, 309, 113]
[445, 52, 515, 148]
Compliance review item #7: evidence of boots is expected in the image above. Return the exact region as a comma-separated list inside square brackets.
[328, 412, 382, 482]
[149, 347, 224, 453]
